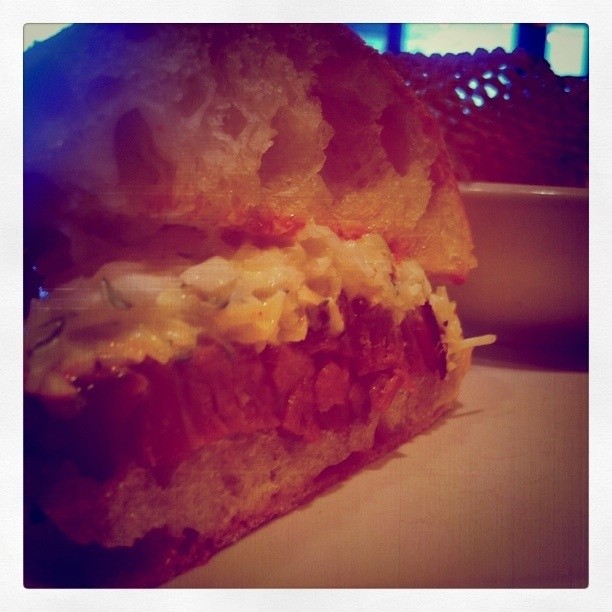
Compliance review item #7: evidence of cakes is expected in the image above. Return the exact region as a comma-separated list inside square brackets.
[26, 27, 481, 584]
